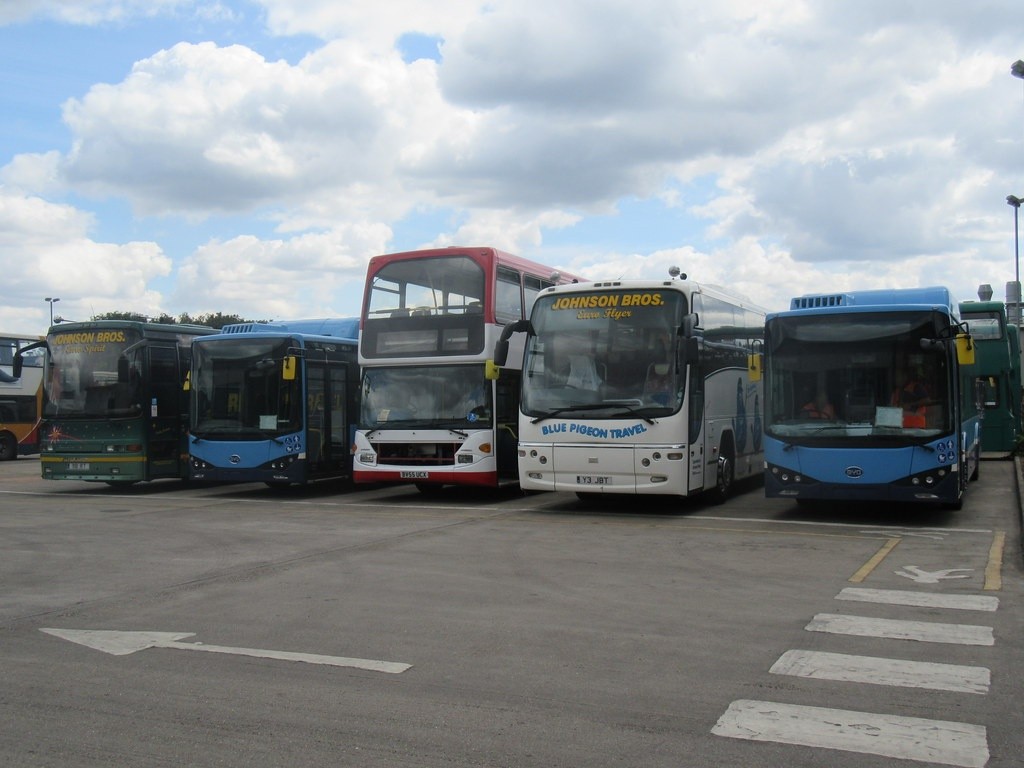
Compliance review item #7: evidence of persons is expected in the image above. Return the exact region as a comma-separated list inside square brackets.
[801, 383, 833, 420]
[891, 367, 931, 410]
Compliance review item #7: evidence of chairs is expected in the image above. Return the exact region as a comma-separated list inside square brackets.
[390, 301, 484, 317]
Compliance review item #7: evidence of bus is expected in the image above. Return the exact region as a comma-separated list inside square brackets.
[494, 265, 795, 507]
[181, 315, 363, 494]
[747, 284, 981, 514]
[13, 319, 221, 490]
[351, 245, 591, 498]
[0, 333, 46, 461]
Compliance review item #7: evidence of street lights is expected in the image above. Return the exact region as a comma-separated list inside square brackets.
[1006, 194, 1024, 351]
[45, 297, 61, 327]
